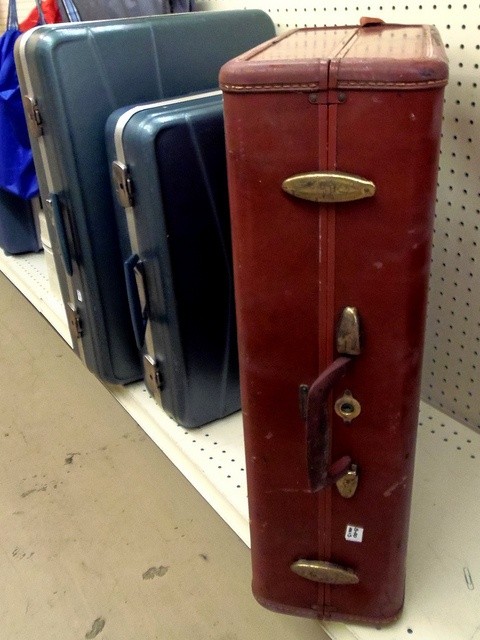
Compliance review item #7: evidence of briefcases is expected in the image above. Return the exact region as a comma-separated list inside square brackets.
[12, 8, 278, 386]
[215, 17, 448, 629]
[103, 89, 242, 429]
